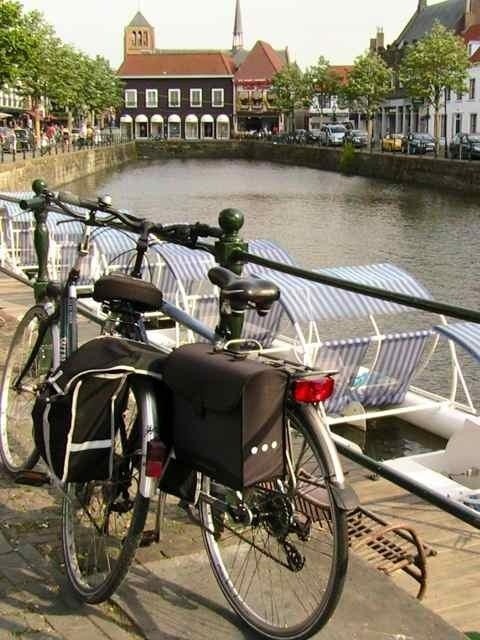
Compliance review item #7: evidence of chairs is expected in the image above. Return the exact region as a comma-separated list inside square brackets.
[192, 292, 433, 415]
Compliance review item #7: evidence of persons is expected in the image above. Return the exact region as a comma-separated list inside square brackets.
[39, 121, 103, 158]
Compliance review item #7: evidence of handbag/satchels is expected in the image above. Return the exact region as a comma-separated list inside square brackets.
[156, 340, 292, 492]
[30, 339, 134, 486]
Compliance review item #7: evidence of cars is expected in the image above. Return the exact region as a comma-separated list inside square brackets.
[288, 129, 321, 144]
[449, 132, 480, 160]
[402, 134, 436, 155]
[0, 127, 34, 153]
[349, 130, 370, 147]
[382, 133, 403, 152]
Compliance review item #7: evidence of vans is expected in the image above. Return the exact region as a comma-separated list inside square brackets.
[320, 125, 347, 146]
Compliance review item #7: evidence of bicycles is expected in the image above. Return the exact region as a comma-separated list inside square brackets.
[0, 189, 173, 604]
[57, 189, 360, 639]
